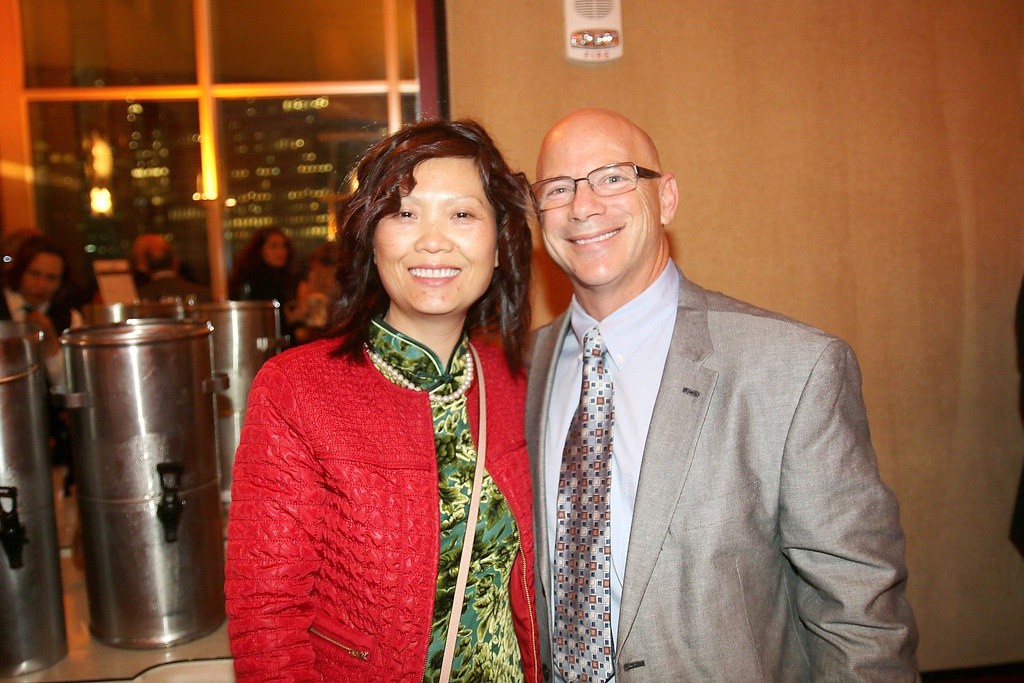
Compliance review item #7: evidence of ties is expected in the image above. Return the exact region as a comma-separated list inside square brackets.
[551, 328, 614, 683]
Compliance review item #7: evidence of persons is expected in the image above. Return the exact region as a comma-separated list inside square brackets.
[522, 106, 924, 683]
[1, 223, 377, 468]
[223, 116, 545, 683]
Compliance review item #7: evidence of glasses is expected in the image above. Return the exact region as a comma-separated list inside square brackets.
[528, 162, 663, 211]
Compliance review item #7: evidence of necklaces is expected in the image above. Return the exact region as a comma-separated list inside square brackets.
[362, 341, 475, 402]
